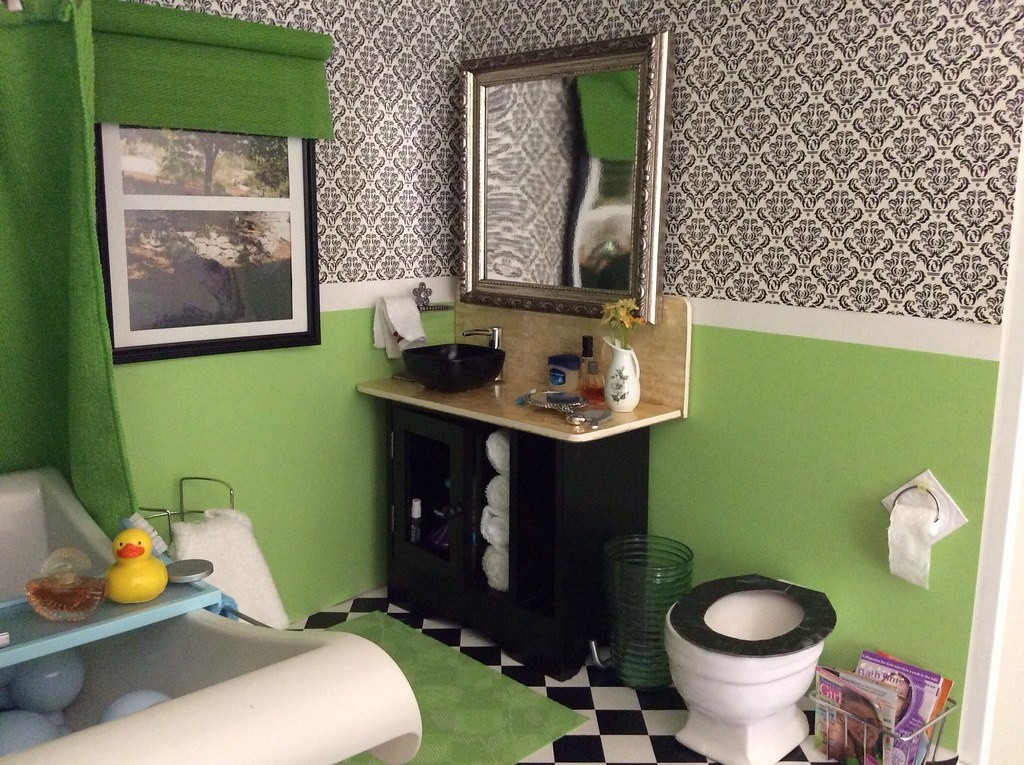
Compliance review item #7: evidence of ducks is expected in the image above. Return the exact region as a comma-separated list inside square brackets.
[103, 529, 168, 604]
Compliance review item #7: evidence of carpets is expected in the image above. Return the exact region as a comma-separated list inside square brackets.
[323, 610, 590, 765]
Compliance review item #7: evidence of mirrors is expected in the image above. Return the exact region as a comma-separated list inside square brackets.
[458, 31, 675, 326]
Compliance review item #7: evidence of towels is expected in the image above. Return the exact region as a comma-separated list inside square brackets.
[168, 506, 290, 633]
[371, 294, 427, 359]
[480, 431, 509, 592]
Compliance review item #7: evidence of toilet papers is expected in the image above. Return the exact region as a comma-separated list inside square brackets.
[887, 505, 942, 591]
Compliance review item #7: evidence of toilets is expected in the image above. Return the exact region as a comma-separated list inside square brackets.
[663, 573, 838, 765]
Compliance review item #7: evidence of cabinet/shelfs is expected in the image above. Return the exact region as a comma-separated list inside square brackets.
[385, 402, 650, 682]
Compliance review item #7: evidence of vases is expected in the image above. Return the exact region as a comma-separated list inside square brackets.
[602, 335, 642, 413]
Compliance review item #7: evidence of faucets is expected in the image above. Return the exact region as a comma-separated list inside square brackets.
[461, 326, 504, 382]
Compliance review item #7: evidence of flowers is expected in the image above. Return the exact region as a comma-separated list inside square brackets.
[601, 299, 647, 350]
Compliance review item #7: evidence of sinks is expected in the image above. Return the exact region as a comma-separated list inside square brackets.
[401, 343, 506, 394]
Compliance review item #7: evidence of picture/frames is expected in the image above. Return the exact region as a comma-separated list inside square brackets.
[92, 121, 322, 364]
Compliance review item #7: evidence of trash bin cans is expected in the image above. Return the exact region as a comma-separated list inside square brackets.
[600, 530, 694, 694]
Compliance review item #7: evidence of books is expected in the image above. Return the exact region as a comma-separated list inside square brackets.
[813, 649, 953, 765]
